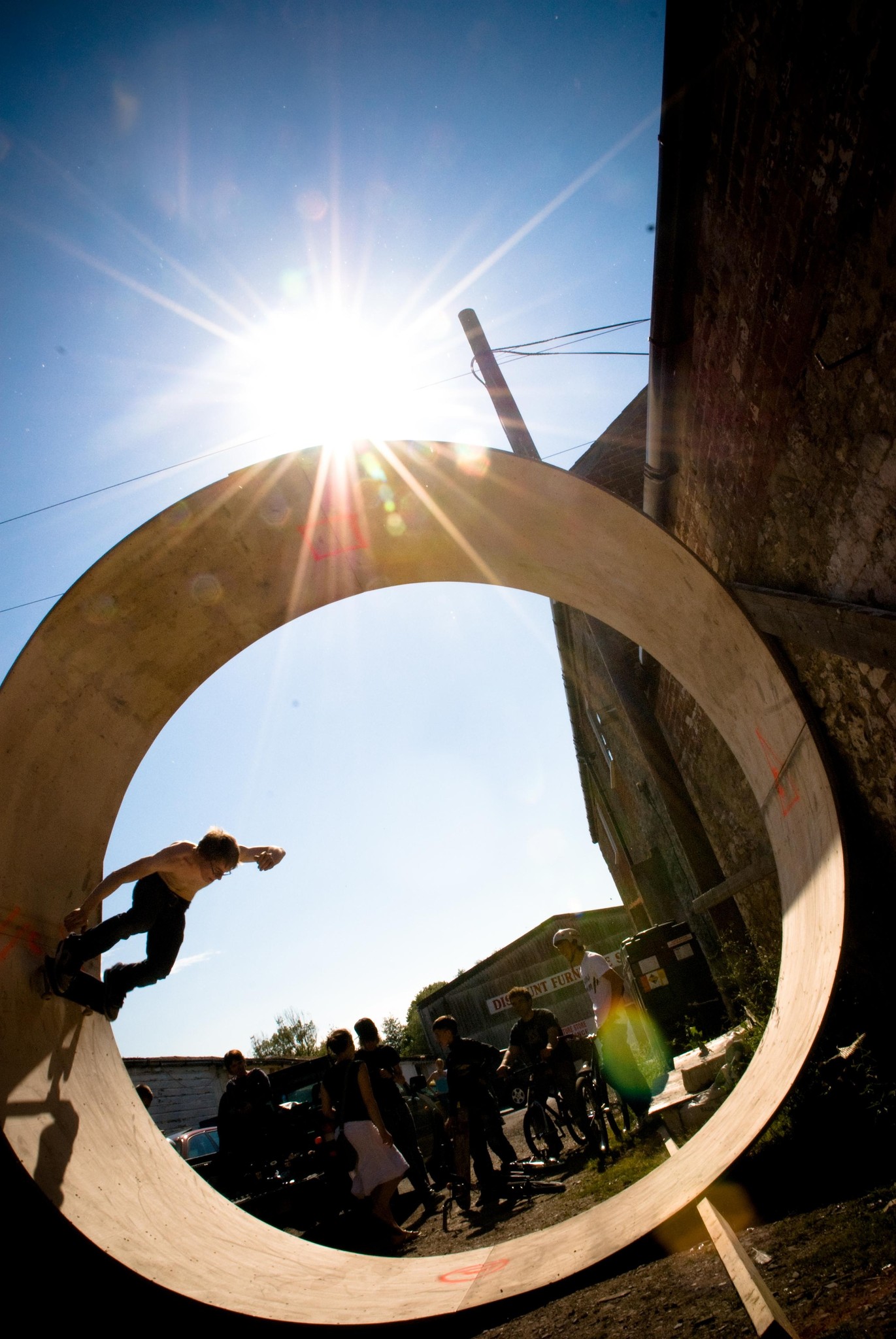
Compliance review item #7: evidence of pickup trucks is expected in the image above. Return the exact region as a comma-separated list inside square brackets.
[492, 1059, 548, 1110]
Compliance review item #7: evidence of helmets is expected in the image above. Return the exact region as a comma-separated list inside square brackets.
[553, 928, 584, 949]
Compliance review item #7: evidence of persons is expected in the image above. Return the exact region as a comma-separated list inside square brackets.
[215, 1050, 276, 1158]
[40, 828, 288, 1020]
[493, 986, 607, 1158]
[351, 1018, 450, 1215]
[319, 1030, 422, 1243]
[553, 928, 656, 1136]
[426, 1058, 451, 1118]
[431, 1014, 524, 1189]
[135, 1084, 154, 1110]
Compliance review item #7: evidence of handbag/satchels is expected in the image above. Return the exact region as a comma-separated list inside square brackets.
[316, 1131, 359, 1176]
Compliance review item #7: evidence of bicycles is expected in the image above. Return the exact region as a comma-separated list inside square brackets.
[443, 1153, 565, 1234]
[493, 1032, 631, 1164]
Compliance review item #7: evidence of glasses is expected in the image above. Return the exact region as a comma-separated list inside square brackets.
[209, 860, 223, 879]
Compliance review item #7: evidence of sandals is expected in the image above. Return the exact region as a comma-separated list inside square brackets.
[374, 1204, 400, 1234]
[394, 1228, 421, 1247]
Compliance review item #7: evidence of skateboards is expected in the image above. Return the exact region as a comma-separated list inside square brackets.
[41, 951, 120, 1024]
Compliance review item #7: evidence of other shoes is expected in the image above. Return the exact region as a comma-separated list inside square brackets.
[421, 1189, 445, 1212]
[104, 968, 123, 1022]
[583, 1129, 602, 1154]
[56, 933, 75, 993]
[475, 1193, 497, 1207]
[630, 1118, 658, 1134]
[546, 1140, 564, 1159]
[500, 1156, 518, 1170]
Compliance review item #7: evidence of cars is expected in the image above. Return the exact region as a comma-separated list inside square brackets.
[167, 1125, 218, 1175]
[394, 1074, 452, 1186]
